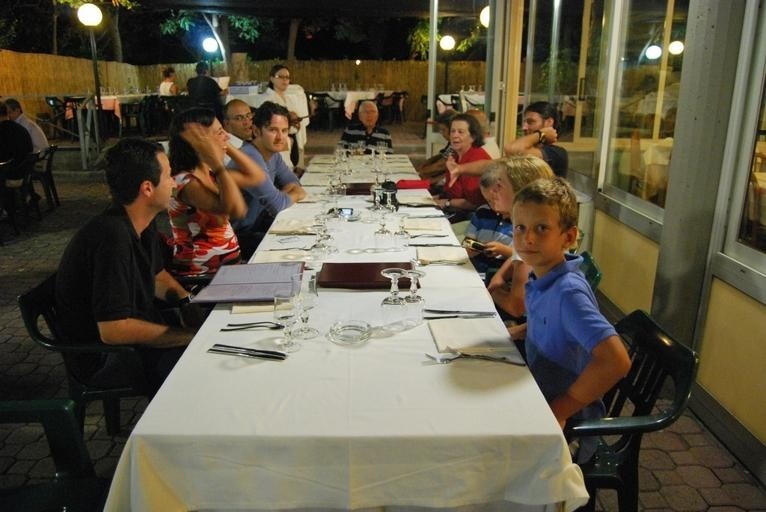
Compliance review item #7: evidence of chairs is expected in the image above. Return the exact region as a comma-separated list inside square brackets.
[305, 87, 405, 127]
[418, 90, 493, 138]
[580, 250, 604, 295]
[558, 306, 702, 511]
[49, 90, 188, 146]
[1, 143, 61, 222]
[2, 397, 103, 510]
[16, 264, 165, 441]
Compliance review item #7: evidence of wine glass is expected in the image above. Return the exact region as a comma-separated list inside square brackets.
[374, 210, 411, 249]
[378, 268, 425, 335]
[311, 200, 337, 261]
[325, 140, 398, 211]
[273, 273, 321, 354]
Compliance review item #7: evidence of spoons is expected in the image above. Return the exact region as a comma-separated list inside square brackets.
[220, 319, 296, 332]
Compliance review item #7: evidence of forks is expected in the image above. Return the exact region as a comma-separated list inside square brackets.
[425, 344, 527, 369]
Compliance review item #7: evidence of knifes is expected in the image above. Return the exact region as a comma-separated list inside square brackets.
[404, 243, 465, 248]
[207, 343, 287, 362]
[424, 309, 499, 320]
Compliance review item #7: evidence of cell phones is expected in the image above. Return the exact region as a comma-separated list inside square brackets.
[328, 207, 354, 217]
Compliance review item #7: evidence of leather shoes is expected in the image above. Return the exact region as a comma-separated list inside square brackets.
[29, 194, 40, 207]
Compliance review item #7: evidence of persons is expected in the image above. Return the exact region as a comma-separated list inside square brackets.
[159, 68, 179, 97]
[340, 101, 392, 156]
[55, 139, 208, 401]
[186, 62, 228, 106]
[218, 64, 305, 259]
[5, 98, 50, 206]
[419, 101, 632, 466]
[0, 101, 32, 220]
[166, 107, 263, 294]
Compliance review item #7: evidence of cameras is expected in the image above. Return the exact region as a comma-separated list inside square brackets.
[461, 236, 485, 253]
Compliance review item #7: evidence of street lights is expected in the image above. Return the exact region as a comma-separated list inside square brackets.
[646, 40, 685, 139]
[76, 3, 106, 151]
[479, 6, 490, 29]
[439, 35, 455, 94]
[203, 37, 218, 78]
